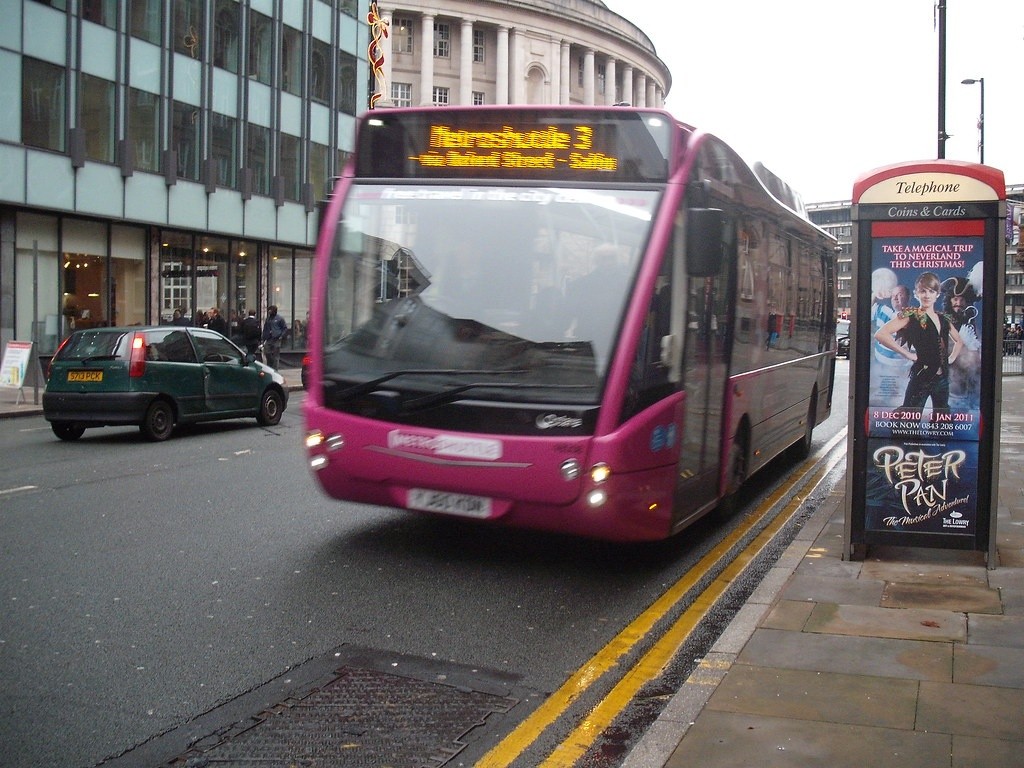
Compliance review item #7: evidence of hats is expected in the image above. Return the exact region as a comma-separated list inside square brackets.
[940, 277, 981, 302]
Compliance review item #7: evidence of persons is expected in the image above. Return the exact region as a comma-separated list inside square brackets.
[765, 307, 777, 350]
[168, 305, 288, 372]
[872, 272, 982, 437]
[1002, 324, 1024, 357]
[290, 312, 310, 349]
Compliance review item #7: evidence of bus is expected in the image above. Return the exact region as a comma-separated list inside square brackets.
[301, 102, 843, 575]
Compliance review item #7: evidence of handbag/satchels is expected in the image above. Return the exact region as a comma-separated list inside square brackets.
[771, 332, 780, 344]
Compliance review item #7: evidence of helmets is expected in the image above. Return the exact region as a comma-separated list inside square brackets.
[872, 268, 898, 305]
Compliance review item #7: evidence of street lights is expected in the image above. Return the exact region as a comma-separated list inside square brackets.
[960, 77, 985, 163]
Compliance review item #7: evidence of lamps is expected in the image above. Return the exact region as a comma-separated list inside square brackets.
[239, 242, 247, 256]
[202, 237, 209, 252]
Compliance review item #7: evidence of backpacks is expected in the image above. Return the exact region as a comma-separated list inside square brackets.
[244, 317, 262, 340]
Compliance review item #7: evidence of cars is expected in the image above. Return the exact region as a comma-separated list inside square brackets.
[41, 325, 291, 443]
[835, 319, 852, 360]
